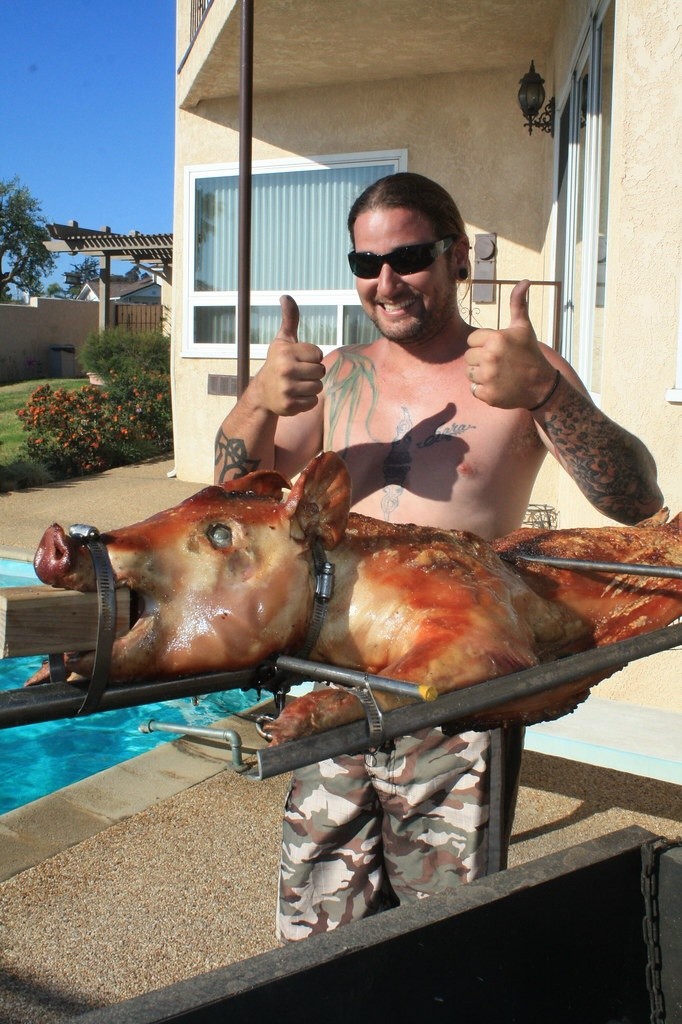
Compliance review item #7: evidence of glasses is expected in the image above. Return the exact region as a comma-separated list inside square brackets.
[347, 232, 458, 279]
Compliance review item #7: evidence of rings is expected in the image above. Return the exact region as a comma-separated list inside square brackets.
[471, 383, 478, 398]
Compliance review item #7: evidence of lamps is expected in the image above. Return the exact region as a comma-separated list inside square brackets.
[517, 60, 556, 138]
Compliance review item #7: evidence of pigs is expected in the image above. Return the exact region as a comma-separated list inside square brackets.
[31, 447, 681, 747]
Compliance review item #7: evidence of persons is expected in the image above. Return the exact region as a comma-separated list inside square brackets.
[213, 173, 664, 950]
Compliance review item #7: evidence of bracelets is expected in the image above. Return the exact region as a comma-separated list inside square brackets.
[529, 370, 560, 410]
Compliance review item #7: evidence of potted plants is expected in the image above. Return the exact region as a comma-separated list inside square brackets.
[78, 327, 127, 386]
